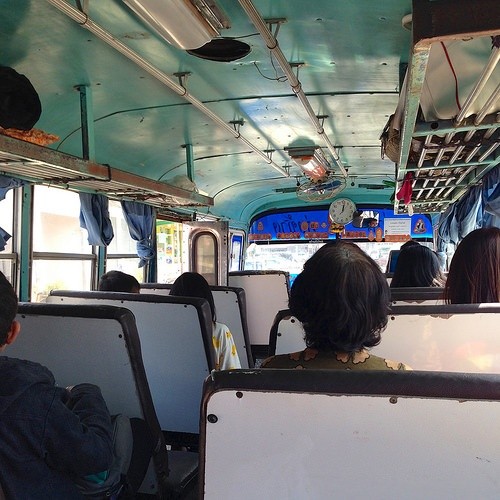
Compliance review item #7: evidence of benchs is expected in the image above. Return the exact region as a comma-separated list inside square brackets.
[0, 270, 500, 500]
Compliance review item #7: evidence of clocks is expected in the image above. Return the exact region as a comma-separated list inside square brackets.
[329, 198, 358, 225]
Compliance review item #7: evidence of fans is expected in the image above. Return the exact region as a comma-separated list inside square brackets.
[296, 176, 347, 202]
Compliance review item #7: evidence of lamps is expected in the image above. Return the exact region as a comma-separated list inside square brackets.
[123, 0, 232, 51]
[284, 146, 336, 184]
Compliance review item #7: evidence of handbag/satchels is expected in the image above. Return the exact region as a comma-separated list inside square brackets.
[380, 119, 467, 162]
[408, 39, 500, 120]
[72, 411, 134, 500]
[0, 64, 42, 132]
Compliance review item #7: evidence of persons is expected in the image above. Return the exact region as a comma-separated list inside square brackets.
[168, 271, 243, 373]
[442, 226, 500, 303]
[0, 268, 157, 500]
[259, 238, 415, 371]
[389, 240, 447, 288]
[98, 270, 141, 293]
